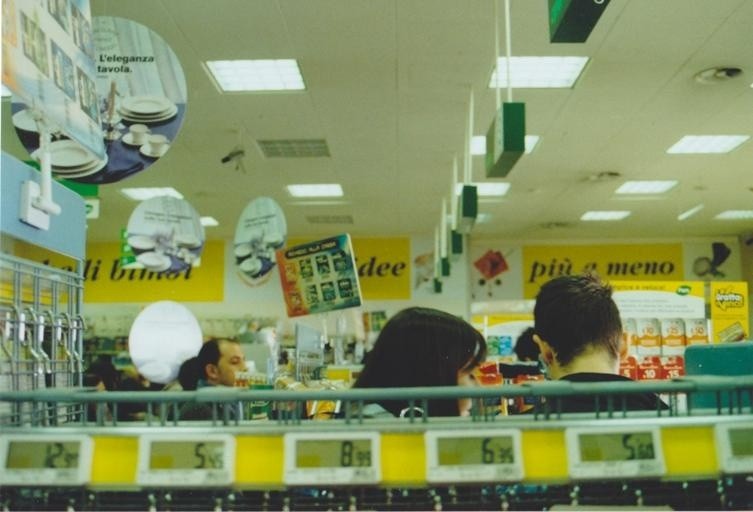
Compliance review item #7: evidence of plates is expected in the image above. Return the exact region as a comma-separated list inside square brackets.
[122, 132, 150, 146]
[140, 144, 169, 157]
[12, 109, 37, 133]
[236, 233, 284, 276]
[116, 94, 179, 123]
[30, 139, 109, 179]
[127, 232, 201, 271]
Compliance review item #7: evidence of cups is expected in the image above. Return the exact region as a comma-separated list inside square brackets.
[130, 124, 152, 144]
[148, 134, 166, 154]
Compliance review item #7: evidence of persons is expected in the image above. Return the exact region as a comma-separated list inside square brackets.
[177, 336, 248, 421]
[510, 325, 546, 369]
[510, 272, 675, 413]
[167, 355, 209, 422]
[341, 305, 489, 418]
[72, 341, 165, 422]
